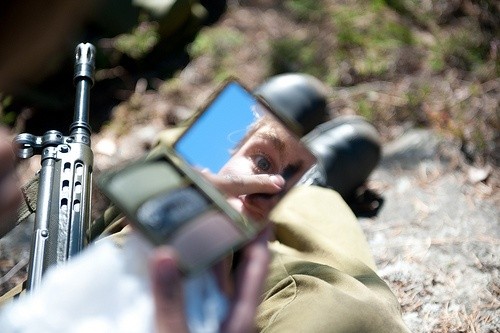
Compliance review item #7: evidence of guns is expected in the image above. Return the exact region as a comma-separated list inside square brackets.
[12, 40, 99, 295]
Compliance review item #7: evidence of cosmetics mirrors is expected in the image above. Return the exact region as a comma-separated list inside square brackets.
[172, 75, 320, 225]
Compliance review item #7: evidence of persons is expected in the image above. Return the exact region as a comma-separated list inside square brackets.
[0, 72, 407, 333]
[195, 115, 312, 223]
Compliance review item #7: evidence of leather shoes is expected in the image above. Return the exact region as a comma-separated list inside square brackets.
[252, 73, 331, 137]
[298, 115, 383, 199]
